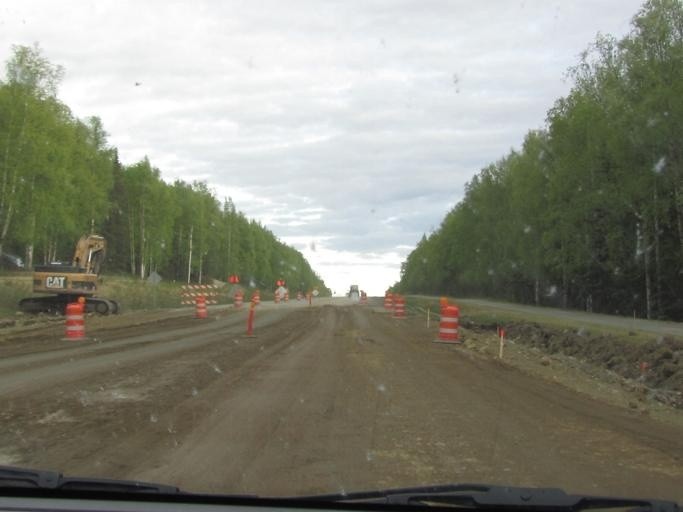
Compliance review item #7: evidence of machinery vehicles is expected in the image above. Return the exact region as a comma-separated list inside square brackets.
[14, 229, 122, 318]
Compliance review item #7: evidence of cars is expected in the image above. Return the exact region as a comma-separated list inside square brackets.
[0, 250, 25, 273]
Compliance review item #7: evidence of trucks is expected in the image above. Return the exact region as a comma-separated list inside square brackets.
[348, 284, 359, 298]
